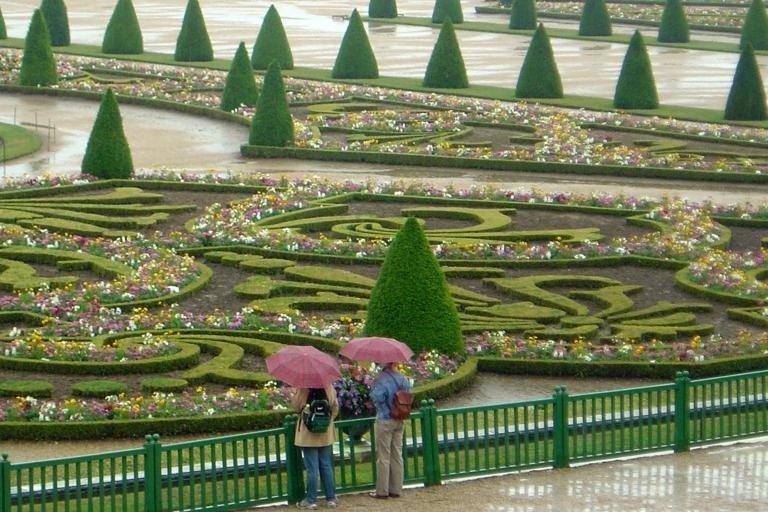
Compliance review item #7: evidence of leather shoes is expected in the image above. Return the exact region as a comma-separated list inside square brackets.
[389, 493, 400, 497]
[369, 492, 388, 498]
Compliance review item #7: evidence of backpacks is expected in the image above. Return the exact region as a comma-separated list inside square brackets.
[383, 373, 413, 420]
[297, 388, 332, 433]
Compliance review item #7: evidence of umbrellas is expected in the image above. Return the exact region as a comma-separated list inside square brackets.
[264, 346, 341, 389]
[338, 336, 416, 364]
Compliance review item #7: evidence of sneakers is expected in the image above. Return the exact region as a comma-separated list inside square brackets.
[327, 495, 339, 508]
[296, 499, 318, 509]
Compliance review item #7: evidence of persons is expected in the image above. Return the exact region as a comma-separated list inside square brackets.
[291, 384, 340, 509]
[368, 363, 410, 499]
